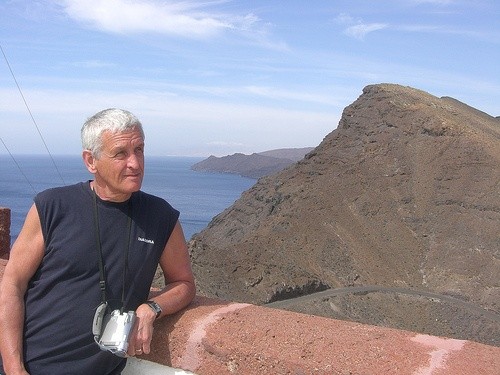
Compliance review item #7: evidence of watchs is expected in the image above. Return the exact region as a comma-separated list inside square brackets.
[141, 300, 162, 318]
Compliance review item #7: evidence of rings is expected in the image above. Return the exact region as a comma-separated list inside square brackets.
[136, 349, 142, 352]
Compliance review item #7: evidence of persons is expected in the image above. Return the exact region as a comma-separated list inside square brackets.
[0, 108, 196, 375]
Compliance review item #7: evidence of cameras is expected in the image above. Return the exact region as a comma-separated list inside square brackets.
[98, 309, 137, 357]
